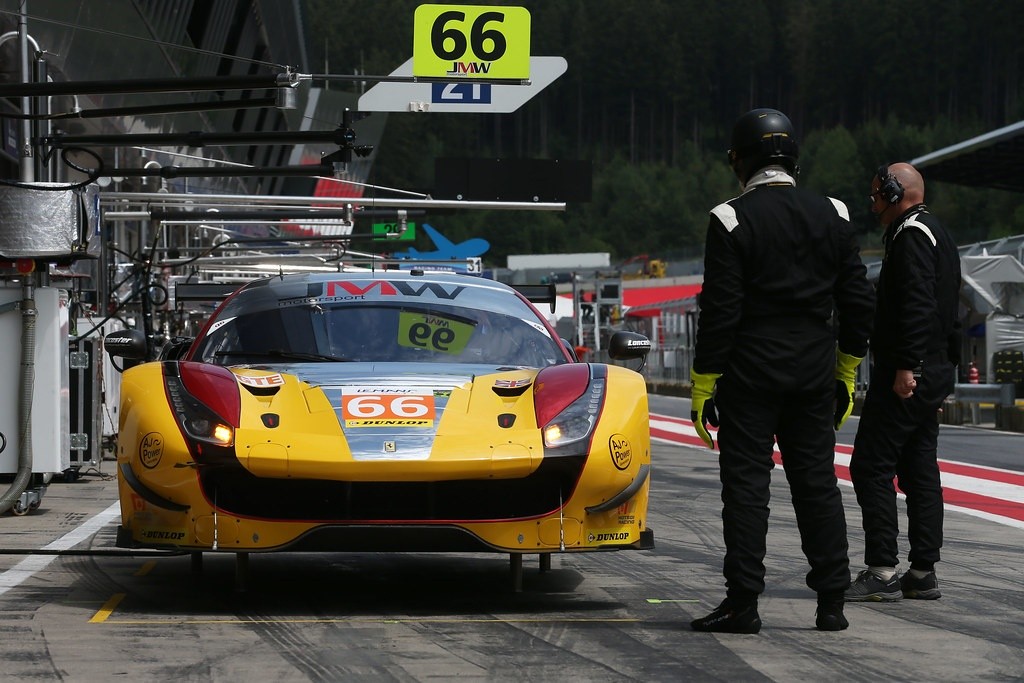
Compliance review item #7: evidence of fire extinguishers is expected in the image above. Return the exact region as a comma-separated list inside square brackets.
[969, 360, 979, 384]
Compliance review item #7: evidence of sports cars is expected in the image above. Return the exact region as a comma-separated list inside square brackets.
[105, 271, 656, 553]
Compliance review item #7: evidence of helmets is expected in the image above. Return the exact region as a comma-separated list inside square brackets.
[731, 108, 796, 156]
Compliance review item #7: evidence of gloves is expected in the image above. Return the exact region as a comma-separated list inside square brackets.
[833, 345, 863, 430]
[690, 369, 719, 450]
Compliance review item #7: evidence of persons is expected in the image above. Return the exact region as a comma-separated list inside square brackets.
[846, 162, 961, 602]
[690, 107, 876, 634]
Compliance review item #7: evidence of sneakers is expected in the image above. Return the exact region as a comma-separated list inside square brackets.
[899, 569, 942, 599]
[842, 570, 903, 601]
[691, 598, 761, 634]
[816, 593, 849, 631]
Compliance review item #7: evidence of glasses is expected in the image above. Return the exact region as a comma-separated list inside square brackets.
[871, 192, 879, 204]
